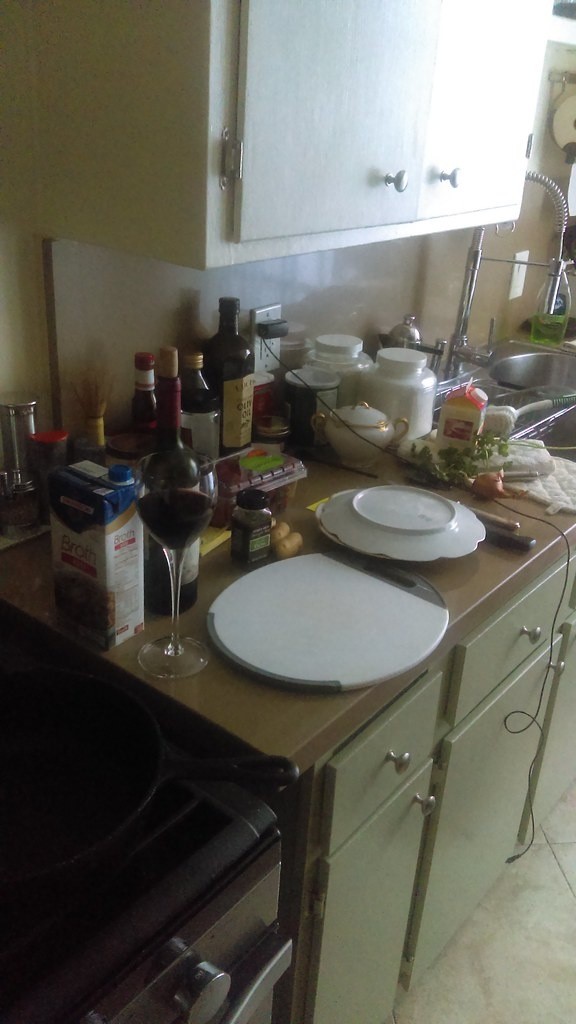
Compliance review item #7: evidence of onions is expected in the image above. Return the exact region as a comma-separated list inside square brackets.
[471, 472, 503, 500]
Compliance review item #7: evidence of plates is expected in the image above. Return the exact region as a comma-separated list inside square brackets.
[321, 484, 486, 562]
[316, 503, 393, 561]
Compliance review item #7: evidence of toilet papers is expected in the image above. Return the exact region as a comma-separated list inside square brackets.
[552, 93, 576, 219]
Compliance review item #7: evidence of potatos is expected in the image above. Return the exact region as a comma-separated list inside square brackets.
[268, 517, 303, 560]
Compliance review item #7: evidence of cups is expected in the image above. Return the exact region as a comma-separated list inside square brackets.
[310, 399, 409, 470]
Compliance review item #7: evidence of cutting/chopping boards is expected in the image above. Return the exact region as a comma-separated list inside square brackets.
[207, 552, 450, 693]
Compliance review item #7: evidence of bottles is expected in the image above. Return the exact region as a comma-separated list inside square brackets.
[198, 298, 256, 458]
[132, 352, 158, 436]
[357, 348, 436, 440]
[180, 350, 220, 462]
[145, 345, 203, 616]
[230, 488, 273, 571]
[301, 335, 373, 407]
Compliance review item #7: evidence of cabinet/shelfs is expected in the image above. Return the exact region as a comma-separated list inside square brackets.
[22, 0, 553, 271]
[282, 550, 574, 1024]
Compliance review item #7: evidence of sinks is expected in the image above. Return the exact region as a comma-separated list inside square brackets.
[412, 375, 556, 445]
[481, 338, 575, 413]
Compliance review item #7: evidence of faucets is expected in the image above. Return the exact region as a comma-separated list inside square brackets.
[443, 170, 569, 372]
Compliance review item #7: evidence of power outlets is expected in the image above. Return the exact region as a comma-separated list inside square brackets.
[250, 303, 281, 374]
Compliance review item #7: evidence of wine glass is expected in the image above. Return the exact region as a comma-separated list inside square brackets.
[132, 449, 219, 678]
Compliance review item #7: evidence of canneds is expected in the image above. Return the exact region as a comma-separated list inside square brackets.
[284, 368, 341, 448]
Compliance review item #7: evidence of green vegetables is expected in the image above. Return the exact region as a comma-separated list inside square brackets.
[403, 432, 576, 486]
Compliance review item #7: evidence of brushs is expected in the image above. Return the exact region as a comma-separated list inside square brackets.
[484, 393, 576, 435]
